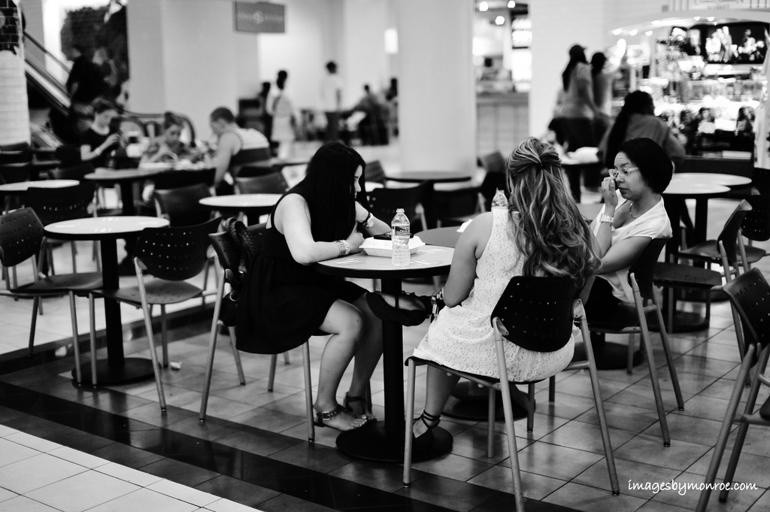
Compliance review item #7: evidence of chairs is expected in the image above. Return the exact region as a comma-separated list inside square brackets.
[0, 138, 769, 512]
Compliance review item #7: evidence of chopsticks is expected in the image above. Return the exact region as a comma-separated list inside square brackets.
[157, 140, 178, 160]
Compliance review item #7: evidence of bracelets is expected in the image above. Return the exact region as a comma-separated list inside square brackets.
[359, 210, 375, 230]
[335, 238, 351, 257]
[598, 213, 616, 222]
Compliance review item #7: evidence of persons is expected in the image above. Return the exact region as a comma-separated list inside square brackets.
[403, 138, 604, 446]
[588, 136, 673, 329]
[546, 44, 755, 207]
[230, 141, 390, 432]
[478, 56, 497, 80]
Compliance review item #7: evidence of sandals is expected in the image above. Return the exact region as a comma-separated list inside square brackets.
[412, 409, 440, 440]
[366, 292, 427, 327]
[312, 393, 368, 431]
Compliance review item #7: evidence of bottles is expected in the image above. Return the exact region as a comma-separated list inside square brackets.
[391, 208, 411, 267]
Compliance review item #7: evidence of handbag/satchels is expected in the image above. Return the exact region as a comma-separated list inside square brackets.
[219, 263, 252, 327]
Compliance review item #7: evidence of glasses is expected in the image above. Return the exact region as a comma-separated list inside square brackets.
[607, 168, 640, 180]
